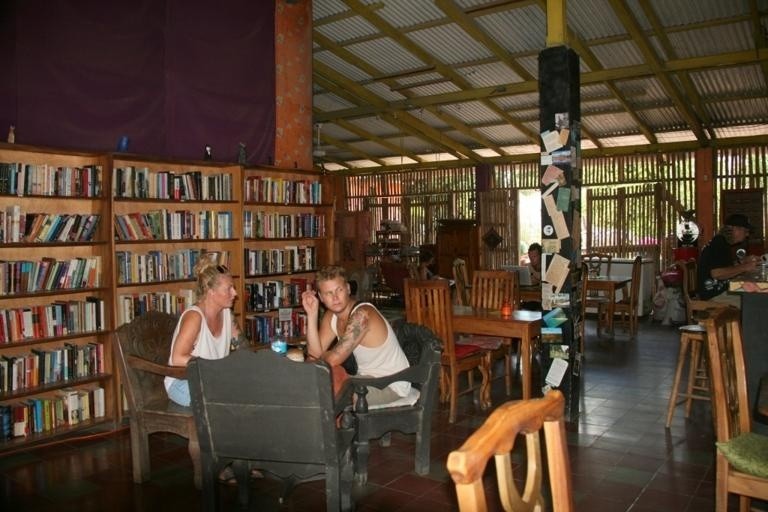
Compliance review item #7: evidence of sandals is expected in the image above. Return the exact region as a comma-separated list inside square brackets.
[217, 473, 238, 486]
[248, 470, 265, 479]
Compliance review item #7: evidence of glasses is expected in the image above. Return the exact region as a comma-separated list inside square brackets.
[201, 264, 229, 288]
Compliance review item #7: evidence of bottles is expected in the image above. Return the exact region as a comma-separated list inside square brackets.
[761, 264, 766, 275]
[270, 328, 287, 359]
[500, 296, 512, 317]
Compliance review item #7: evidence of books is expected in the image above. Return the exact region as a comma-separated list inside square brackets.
[1, 161, 107, 440]
[245, 177, 326, 344]
[113, 166, 233, 411]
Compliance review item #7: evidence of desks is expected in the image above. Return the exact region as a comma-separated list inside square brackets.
[403, 305, 545, 401]
[724, 250, 767, 437]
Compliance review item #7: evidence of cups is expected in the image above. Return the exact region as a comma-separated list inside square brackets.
[760, 253, 768, 265]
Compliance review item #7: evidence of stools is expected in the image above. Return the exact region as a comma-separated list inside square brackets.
[665, 323, 711, 428]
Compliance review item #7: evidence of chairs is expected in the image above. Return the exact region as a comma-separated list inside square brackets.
[401, 277, 488, 425]
[705, 302, 767, 511]
[445, 389, 575, 512]
[460, 269, 521, 394]
[679, 256, 718, 323]
[376, 254, 645, 340]
[183, 348, 357, 512]
[110, 311, 207, 490]
[331, 321, 443, 476]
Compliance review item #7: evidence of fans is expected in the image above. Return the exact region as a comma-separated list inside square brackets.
[312, 121, 338, 158]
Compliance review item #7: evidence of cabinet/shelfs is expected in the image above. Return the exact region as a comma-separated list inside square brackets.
[0, 145, 120, 455]
[94, 162, 244, 442]
[435, 225, 480, 283]
[372, 229, 409, 295]
[225, 164, 338, 353]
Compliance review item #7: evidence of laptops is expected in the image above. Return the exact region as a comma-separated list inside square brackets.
[503, 265, 539, 287]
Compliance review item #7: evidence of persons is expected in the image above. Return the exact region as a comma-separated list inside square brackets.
[526, 242, 541, 285]
[696, 213, 758, 309]
[301, 264, 413, 428]
[418, 250, 440, 280]
[165, 257, 264, 486]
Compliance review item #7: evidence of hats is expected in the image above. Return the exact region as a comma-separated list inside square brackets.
[725, 214, 754, 229]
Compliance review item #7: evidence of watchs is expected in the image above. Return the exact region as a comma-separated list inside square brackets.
[530, 270, 536, 275]
